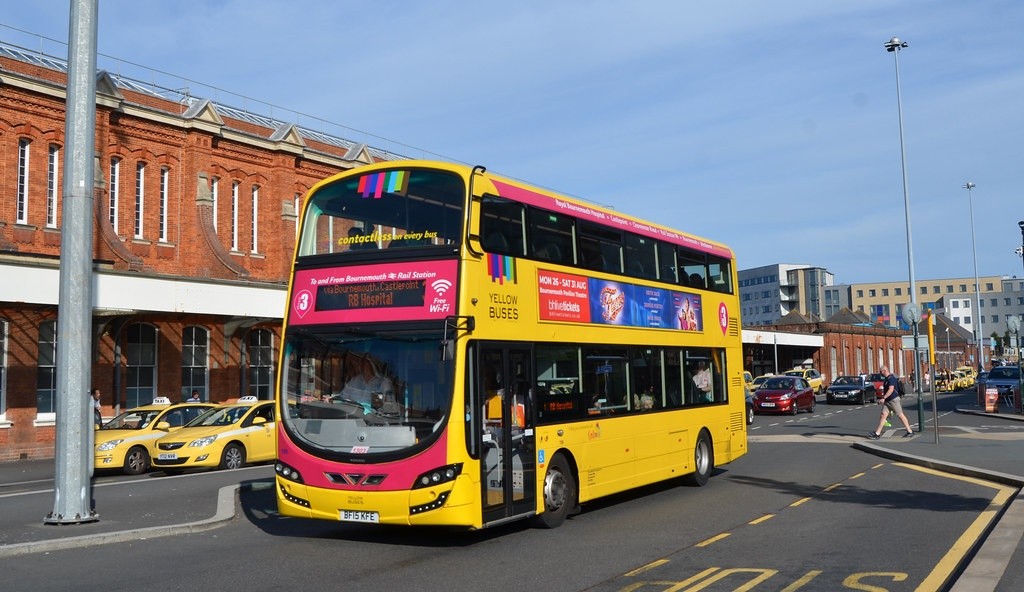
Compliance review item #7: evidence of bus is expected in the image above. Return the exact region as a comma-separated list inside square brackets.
[272, 158, 752, 534]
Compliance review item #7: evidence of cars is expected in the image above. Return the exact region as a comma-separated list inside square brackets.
[94, 396, 223, 476]
[864, 372, 899, 400]
[784, 368, 824, 395]
[825, 374, 877, 405]
[150, 394, 298, 474]
[934, 365, 1024, 403]
[752, 374, 817, 416]
[743, 370, 785, 425]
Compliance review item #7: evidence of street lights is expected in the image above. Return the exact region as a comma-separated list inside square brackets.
[962, 182, 985, 375]
[883, 35, 926, 432]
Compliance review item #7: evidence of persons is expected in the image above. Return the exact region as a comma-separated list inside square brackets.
[536, 232, 552, 259]
[687, 317, 697, 332]
[866, 365, 914, 439]
[324, 350, 393, 410]
[91, 387, 103, 419]
[341, 224, 379, 251]
[185, 388, 203, 403]
[924, 371, 930, 388]
[689, 308, 696, 320]
[691, 348, 712, 401]
[910, 368, 917, 393]
[674, 262, 690, 281]
[610, 385, 655, 409]
[937, 366, 953, 393]
[675, 309, 687, 332]
[684, 296, 691, 318]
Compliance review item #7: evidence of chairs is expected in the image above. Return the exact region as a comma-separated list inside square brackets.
[388, 230, 716, 290]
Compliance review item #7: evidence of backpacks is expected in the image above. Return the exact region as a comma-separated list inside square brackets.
[888, 374, 905, 397]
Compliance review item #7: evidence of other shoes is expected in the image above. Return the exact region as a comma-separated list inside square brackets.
[903, 430, 915, 437]
[865, 431, 880, 440]
